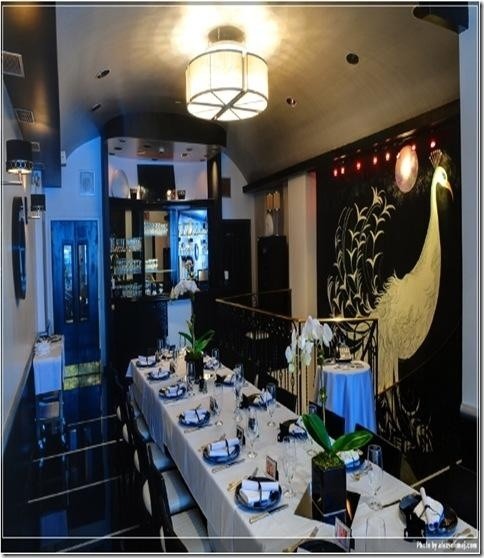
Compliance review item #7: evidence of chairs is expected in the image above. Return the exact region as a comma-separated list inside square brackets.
[110, 366, 214, 552]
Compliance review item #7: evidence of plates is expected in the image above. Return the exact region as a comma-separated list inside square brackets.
[135, 344, 362, 552]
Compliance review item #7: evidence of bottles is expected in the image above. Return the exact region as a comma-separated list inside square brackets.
[111, 184, 210, 300]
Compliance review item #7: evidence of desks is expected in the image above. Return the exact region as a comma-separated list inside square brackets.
[31, 334, 64, 444]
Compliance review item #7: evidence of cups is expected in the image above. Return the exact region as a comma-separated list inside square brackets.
[365, 516, 386, 551]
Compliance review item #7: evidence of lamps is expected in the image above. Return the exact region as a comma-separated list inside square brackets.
[27, 194, 46, 219]
[183, 25, 271, 124]
[3, 137, 33, 188]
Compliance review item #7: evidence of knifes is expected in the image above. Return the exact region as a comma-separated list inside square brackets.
[446, 528, 474, 552]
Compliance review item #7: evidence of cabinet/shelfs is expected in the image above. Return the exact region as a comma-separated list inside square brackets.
[108, 194, 213, 362]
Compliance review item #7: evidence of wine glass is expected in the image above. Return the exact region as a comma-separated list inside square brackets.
[366, 444, 383, 512]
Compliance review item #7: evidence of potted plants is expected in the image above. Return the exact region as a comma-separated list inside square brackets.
[177, 319, 216, 382]
